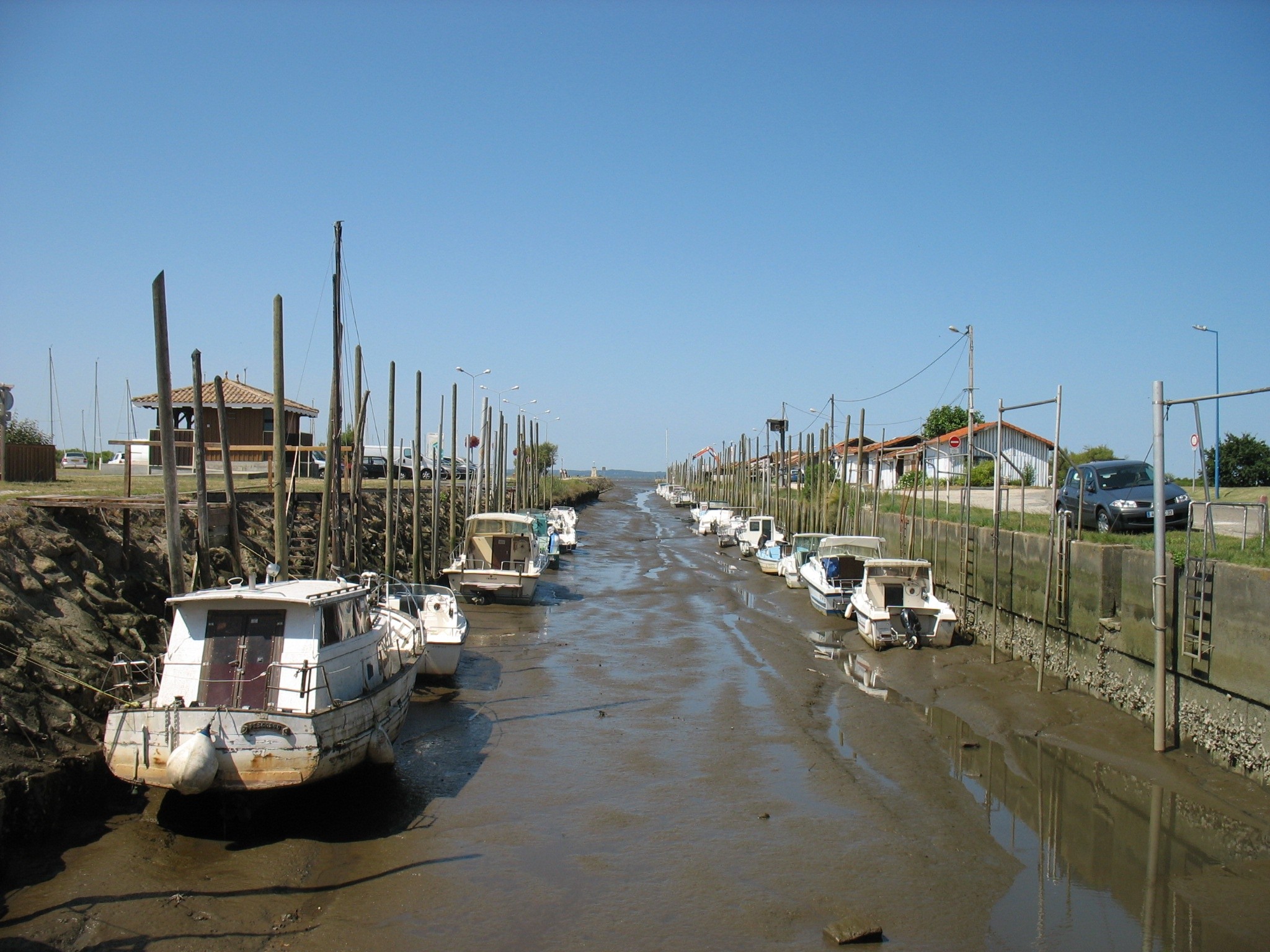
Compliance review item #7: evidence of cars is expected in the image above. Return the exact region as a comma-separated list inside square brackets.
[62, 452, 89, 469]
[787, 470, 805, 483]
[1054, 458, 1194, 534]
[313, 450, 345, 479]
[347, 456, 413, 480]
[435, 456, 486, 481]
[108, 452, 125, 464]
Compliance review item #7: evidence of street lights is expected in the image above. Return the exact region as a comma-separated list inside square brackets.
[503, 399, 562, 504]
[949, 324, 969, 629]
[480, 385, 519, 509]
[1194, 324, 1220, 497]
[456, 366, 491, 531]
[752, 420, 770, 456]
[809, 394, 835, 480]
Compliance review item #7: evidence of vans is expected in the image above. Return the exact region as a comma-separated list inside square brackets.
[364, 445, 437, 480]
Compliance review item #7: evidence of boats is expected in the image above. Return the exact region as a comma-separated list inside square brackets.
[810, 631, 889, 701]
[803, 535, 887, 618]
[845, 558, 957, 652]
[654, 483, 792, 576]
[360, 571, 468, 676]
[442, 513, 551, 604]
[520, 508, 563, 569]
[551, 506, 580, 529]
[100, 564, 425, 795]
[543, 512, 577, 552]
[776, 533, 840, 589]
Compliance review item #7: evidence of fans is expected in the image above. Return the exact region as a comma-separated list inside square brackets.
[516, 540, 529, 552]
